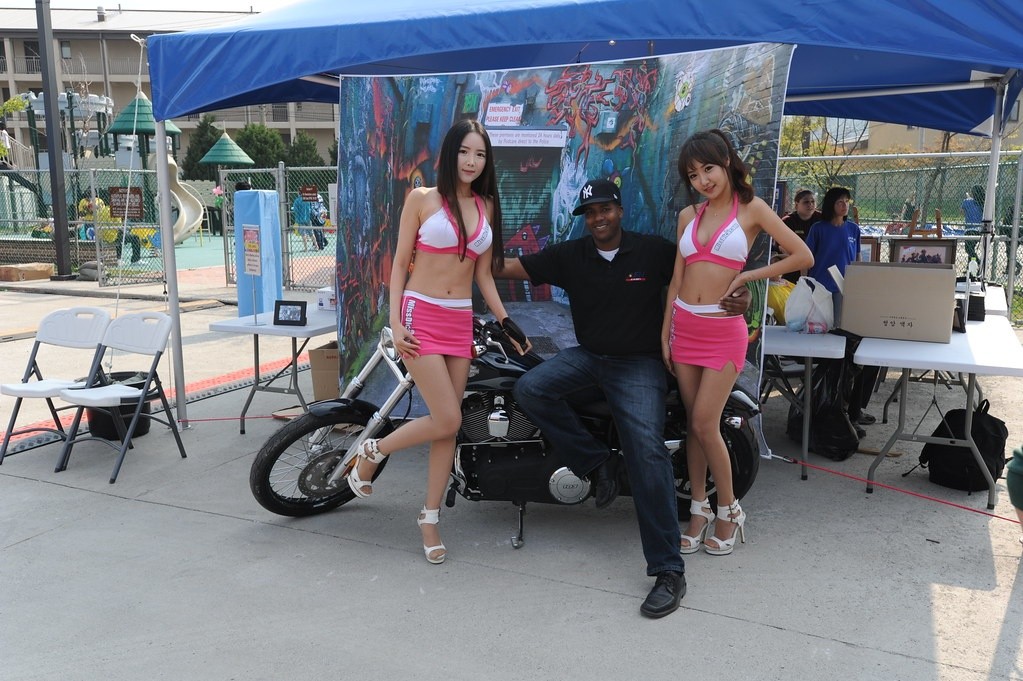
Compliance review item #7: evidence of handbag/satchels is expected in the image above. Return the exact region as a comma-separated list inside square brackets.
[784, 276, 835, 333]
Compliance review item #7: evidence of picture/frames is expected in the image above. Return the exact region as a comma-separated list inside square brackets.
[953, 306, 965, 332]
[860, 239, 958, 265]
[773, 180, 788, 219]
[274, 300, 307, 326]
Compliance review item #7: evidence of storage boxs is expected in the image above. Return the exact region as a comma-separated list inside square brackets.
[318, 288, 336, 310]
[308, 341, 339, 400]
[827, 261, 956, 345]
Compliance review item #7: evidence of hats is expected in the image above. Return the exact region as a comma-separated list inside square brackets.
[572, 179, 621, 216]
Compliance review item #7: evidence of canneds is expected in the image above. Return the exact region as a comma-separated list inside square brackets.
[804, 322, 828, 333]
[788, 320, 805, 332]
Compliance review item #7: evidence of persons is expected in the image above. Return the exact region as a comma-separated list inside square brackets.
[961, 184, 986, 276]
[767, 250, 880, 439]
[800, 187, 862, 289]
[490, 178, 752, 620]
[901, 191, 916, 219]
[348, 120, 532, 564]
[999, 205, 1023, 276]
[901, 249, 942, 263]
[662, 129, 815, 555]
[770, 190, 822, 365]
[884, 213, 904, 251]
[293, 186, 329, 252]
[78, 186, 107, 239]
[115, 230, 149, 266]
[235, 180, 252, 191]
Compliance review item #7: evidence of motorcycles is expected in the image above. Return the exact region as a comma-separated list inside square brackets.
[250, 312, 762, 550]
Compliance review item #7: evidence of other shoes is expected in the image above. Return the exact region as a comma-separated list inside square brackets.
[851, 422, 866, 438]
[854, 411, 876, 424]
[133, 259, 149, 266]
[117, 258, 125, 265]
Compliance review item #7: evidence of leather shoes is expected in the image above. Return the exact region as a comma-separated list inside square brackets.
[640, 570, 686, 617]
[593, 451, 623, 509]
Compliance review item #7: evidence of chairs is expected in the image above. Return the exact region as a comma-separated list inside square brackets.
[0, 308, 188, 483]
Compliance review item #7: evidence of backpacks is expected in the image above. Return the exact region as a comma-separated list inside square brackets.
[919, 398, 1009, 491]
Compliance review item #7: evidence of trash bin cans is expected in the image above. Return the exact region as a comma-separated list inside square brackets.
[74, 371, 151, 440]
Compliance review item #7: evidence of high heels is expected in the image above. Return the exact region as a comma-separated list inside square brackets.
[704, 499, 746, 555]
[344, 439, 386, 500]
[680, 497, 714, 553]
[416, 504, 446, 564]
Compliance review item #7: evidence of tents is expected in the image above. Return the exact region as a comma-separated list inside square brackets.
[146, 0, 1023, 431]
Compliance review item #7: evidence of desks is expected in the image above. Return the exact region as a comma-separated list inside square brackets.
[207, 304, 338, 434]
[880, 284, 1007, 424]
[763, 324, 846, 479]
[854, 314, 1023, 509]
